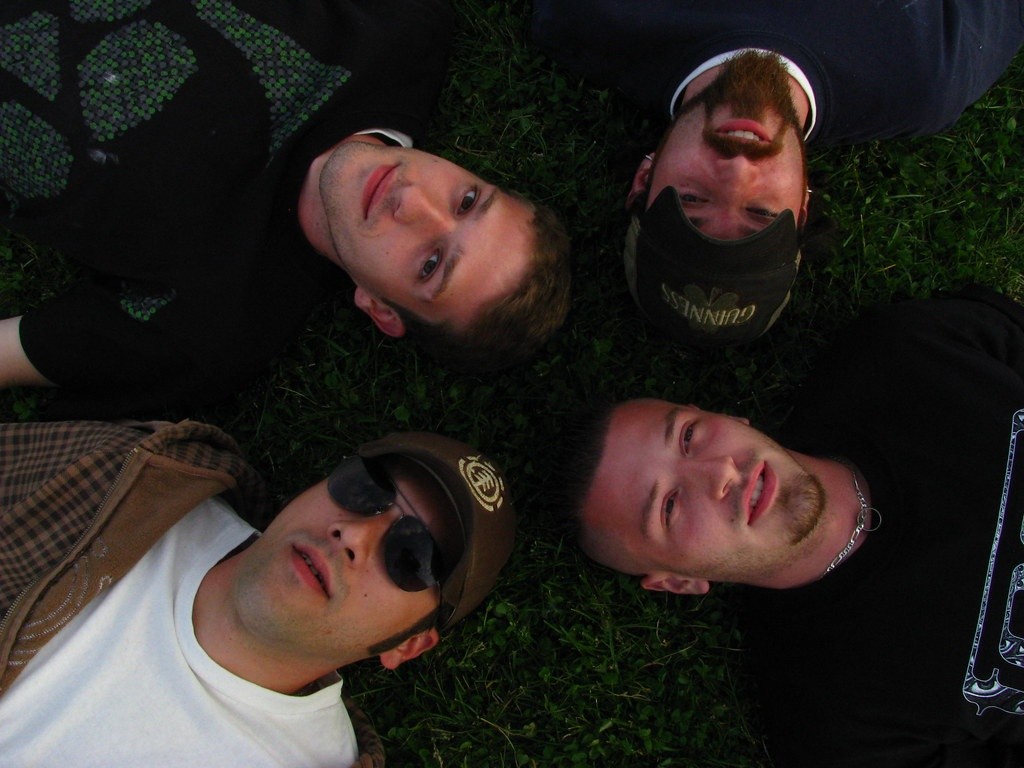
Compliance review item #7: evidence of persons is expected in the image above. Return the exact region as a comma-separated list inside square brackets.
[0, 418, 516, 768]
[546, 286, 1024, 768]
[527, 0, 1024, 344]
[0, 0, 574, 416]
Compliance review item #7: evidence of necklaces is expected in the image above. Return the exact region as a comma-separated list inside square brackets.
[821, 454, 882, 576]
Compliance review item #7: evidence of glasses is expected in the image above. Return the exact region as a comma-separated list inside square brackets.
[327, 454, 447, 612]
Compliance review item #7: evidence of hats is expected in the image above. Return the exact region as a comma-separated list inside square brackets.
[358, 430, 515, 634]
[621, 185, 802, 351]
